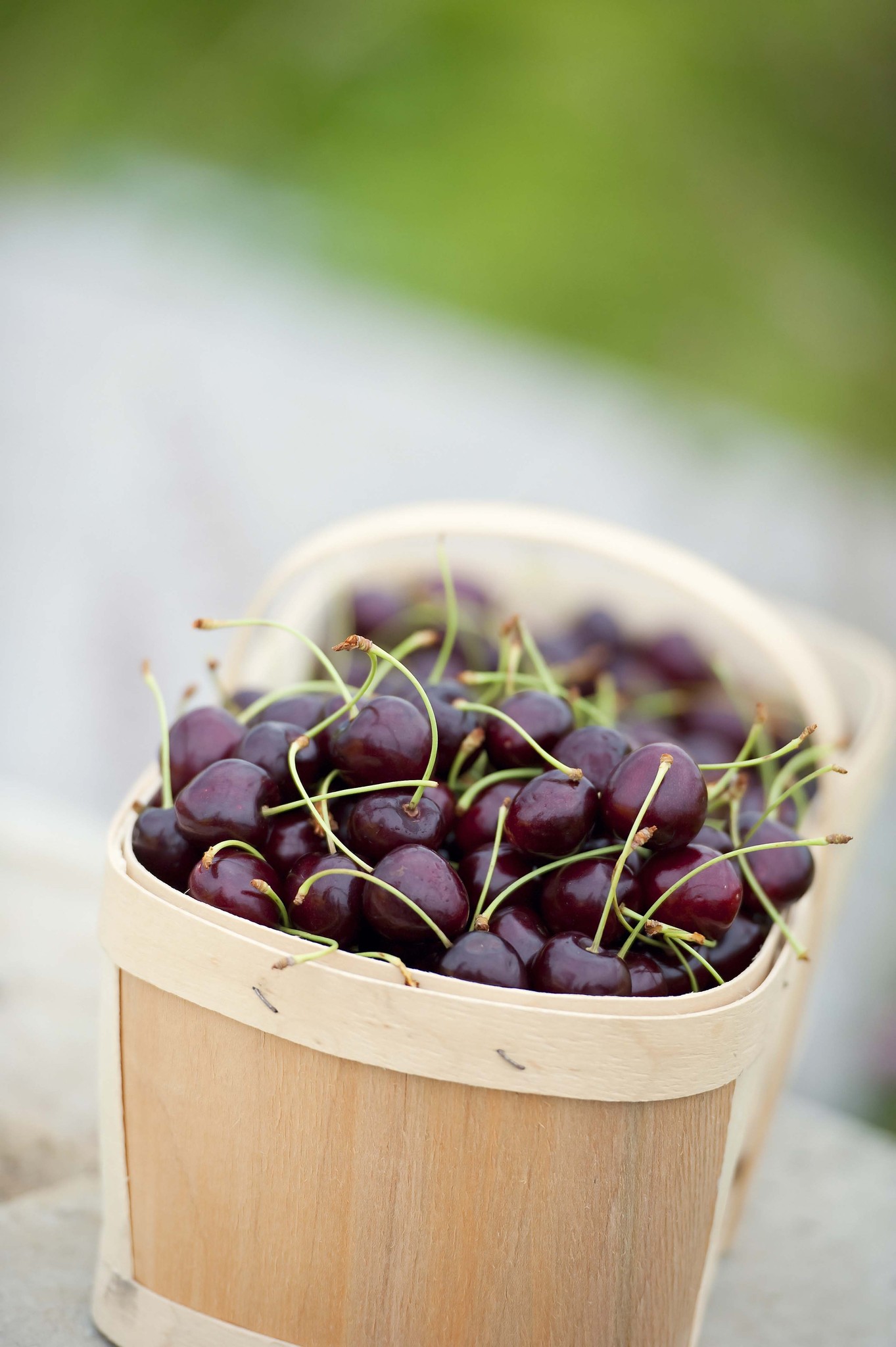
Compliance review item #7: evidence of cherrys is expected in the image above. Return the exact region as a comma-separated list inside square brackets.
[131, 540, 855, 995]
